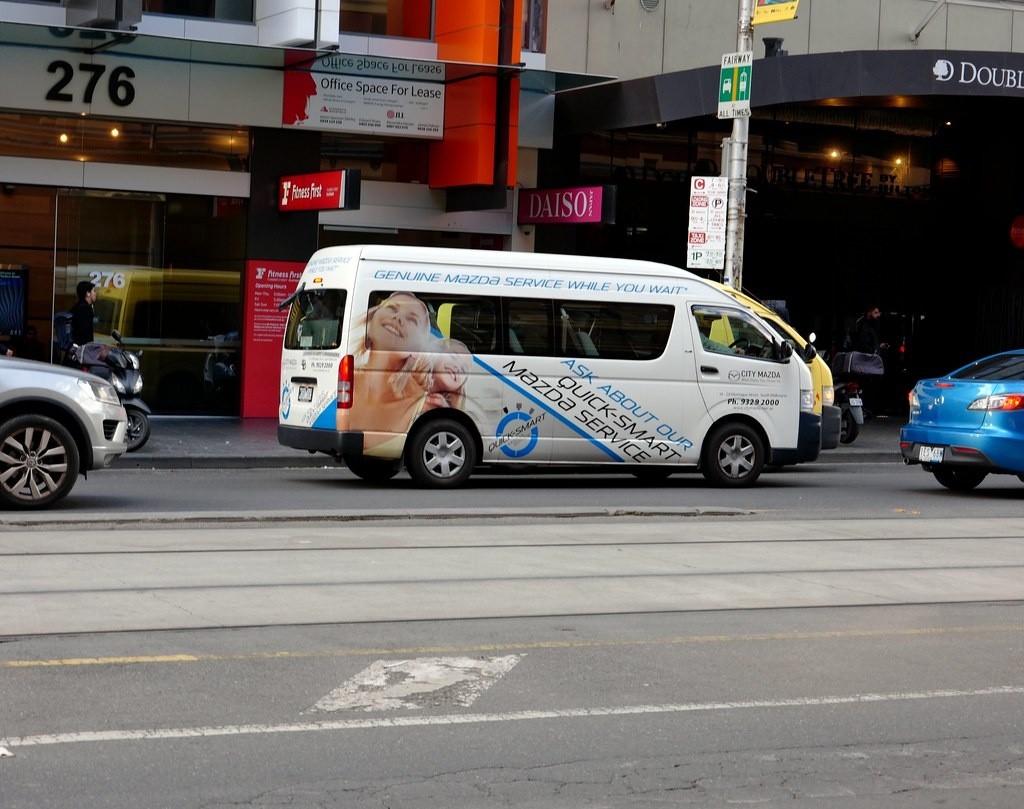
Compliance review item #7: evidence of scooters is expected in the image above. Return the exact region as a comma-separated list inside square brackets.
[809, 332, 884, 444]
[69, 330, 152, 451]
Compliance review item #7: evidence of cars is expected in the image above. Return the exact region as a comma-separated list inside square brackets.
[900, 349, 1024, 490]
[0, 356, 128, 510]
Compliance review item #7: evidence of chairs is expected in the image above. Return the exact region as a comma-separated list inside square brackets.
[578, 332, 599, 356]
[507, 329, 525, 354]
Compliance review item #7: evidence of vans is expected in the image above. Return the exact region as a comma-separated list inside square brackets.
[277, 246, 822, 488]
[436, 279, 842, 471]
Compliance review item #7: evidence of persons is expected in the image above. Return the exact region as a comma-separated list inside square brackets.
[694, 310, 746, 356]
[62, 281, 96, 371]
[335, 291, 472, 457]
[845, 306, 890, 355]
